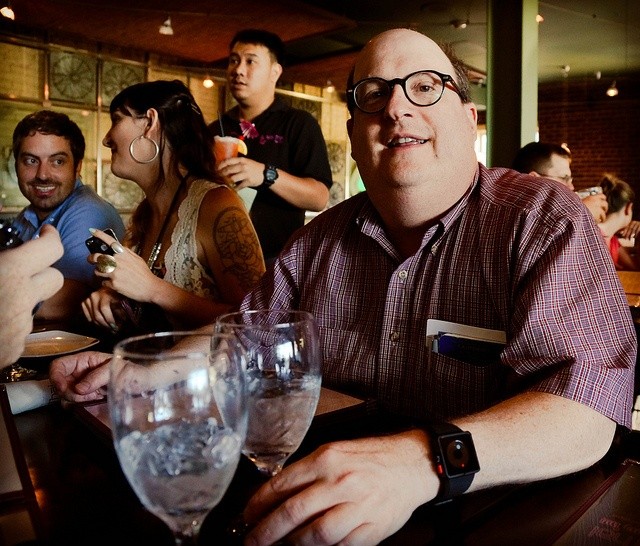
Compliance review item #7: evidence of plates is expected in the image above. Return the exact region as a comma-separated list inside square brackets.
[21, 331, 99, 358]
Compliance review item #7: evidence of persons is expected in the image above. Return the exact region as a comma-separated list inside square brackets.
[79, 78, 268, 334]
[0, 109, 130, 323]
[48, 27, 638, 545]
[512, 139, 610, 224]
[206, 27, 336, 270]
[1, 221, 68, 376]
[596, 172, 639, 273]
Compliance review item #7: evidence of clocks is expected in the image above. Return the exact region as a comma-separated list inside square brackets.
[46, 42, 96, 104]
[326, 146, 346, 207]
[102, 165, 142, 207]
[102, 60, 147, 108]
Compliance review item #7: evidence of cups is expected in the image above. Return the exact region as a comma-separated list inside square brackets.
[213, 137, 239, 183]
[574, 187, 603, 202]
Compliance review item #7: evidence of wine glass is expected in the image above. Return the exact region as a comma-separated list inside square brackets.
[109, 331, 248, 546]
[212, 309, 323, 480]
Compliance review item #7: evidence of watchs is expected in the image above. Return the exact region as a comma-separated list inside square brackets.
[417, 412, 477, 509]
[259, 161, 275, 196]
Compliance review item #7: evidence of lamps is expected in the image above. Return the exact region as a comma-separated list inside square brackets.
[455, 19, 469, 32]
[157, 15, 174, 39]
[607, 81, 619, 100]
[327, 80, 333, 97]
[0, 4, 17, 21]
[202, 73, 215, 88]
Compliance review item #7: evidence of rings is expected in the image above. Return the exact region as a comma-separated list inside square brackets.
[112, 242, 121, 254]
[96, 254, 116, 275]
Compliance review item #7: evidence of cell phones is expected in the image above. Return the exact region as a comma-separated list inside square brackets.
[85, 228, 122, 255]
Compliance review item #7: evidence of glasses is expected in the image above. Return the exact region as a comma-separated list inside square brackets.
[345, 69, 466, 115]
[541, 170, 575, 185]
